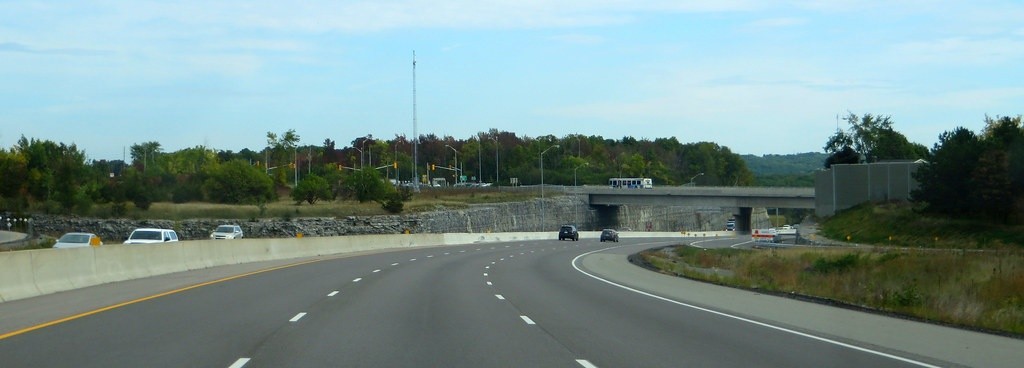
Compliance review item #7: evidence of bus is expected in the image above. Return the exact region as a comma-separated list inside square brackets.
[608, 177, 652, 189]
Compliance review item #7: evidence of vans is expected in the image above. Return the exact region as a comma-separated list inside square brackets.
[121, 228, 178, 244]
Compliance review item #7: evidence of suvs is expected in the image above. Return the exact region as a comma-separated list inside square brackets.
[210, 224, 244, 241]
[600, 229, 619, 242]
[558, 225, 578, 241]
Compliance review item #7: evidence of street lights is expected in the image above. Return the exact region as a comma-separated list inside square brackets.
[445, 144, 458, 187]
[540, 144, 560, 231]
[352, 147, 362, 175]
[395, 140, 401, 185]
[363, 136, 373, 173]
[574, 162, 590, 228]
[488, 135, 498, 182]
[690, 173, 704, 186]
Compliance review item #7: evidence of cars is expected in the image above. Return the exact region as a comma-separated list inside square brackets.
[727, 218, 736, 231]
[783, 225, 790, 229]
[53, 233, 103, 247]
